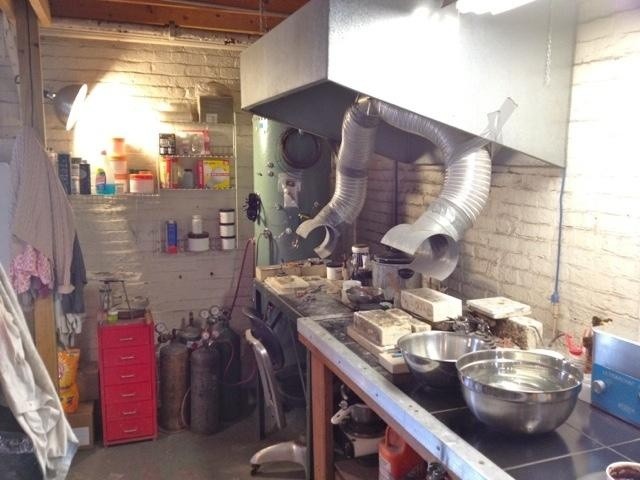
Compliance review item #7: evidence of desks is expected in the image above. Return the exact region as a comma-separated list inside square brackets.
[255, 277, 396, 440]
[297, 314, 639, 480]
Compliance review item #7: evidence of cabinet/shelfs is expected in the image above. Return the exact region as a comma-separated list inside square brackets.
[157, 112, 239, 256]
[97, 313, 158, 449]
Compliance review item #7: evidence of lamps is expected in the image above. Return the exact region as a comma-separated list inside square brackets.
[43, 82, 89, 131]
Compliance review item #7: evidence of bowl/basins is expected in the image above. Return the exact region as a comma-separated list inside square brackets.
[455, 349, 584, 434]
[347, 286, 383, 304]
[397, 330, 488, 387]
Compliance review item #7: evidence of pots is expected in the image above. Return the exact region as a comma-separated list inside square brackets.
[331, 384, 377, 427]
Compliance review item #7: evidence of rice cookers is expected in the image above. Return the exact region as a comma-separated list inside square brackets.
[370, 247, 422, 289]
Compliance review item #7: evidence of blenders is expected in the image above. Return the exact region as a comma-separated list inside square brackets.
[350, 244, 369, 282]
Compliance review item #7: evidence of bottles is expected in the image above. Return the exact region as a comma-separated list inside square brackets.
[183, 168, 194, 189]
[325, 263, 343, 280]
[191, 214, 202, 234]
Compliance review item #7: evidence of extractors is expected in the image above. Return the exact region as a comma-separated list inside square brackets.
[237, 0, 577, 282]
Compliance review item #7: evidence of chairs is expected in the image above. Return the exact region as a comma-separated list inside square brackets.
[241, 305, 309, 480]
